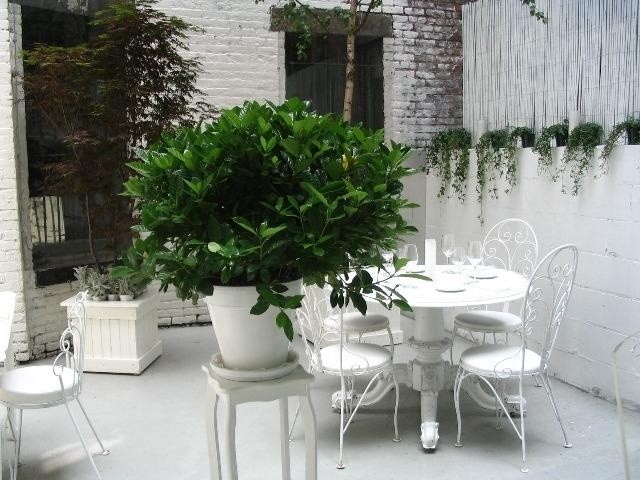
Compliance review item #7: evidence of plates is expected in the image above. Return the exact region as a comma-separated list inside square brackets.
[412, 269, 424, 273]
[436, 283, 463, 293]
[471, 273, 500, 278]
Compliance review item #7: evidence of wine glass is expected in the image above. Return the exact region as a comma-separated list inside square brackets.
[441, 232, 466, 281]
[402, 244, 418, 274]
[467, 241, 483, 286]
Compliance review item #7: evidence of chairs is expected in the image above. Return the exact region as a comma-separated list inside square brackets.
[288, 281, 401, 470]
[450, 216, 543, 389]
[301, 278, 395, 391]
[1, 289, 110, 480]
[452, 243, 581, 473]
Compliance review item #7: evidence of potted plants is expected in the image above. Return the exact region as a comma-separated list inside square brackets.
[426, 117, 638, 224]
[73, 264, 148, 302]
[112, 96, 433, 382]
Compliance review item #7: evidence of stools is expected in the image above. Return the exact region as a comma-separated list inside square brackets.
[202, 362, 320, 480]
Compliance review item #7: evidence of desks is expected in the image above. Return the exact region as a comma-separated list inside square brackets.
[330, 264, 533, 450]
